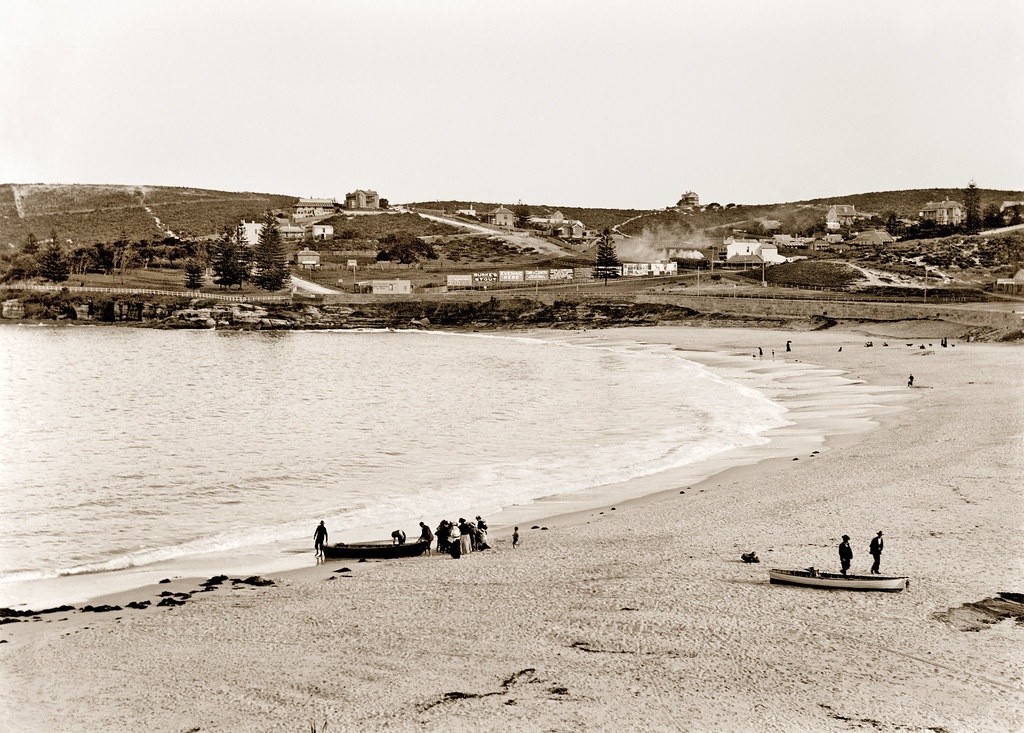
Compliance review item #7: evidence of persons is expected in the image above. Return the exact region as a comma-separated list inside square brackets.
[417, 521, 434, 557]
[391, 529, 407, 547]
[907, 373, 915, 386]
[434, 515, 492, 559]
[838, 534, 854, 575]
[512, 526, 520, 548]
[868, 530, 885, 574]
[771, 348, 775, 357]
[313, 520, 328, 556]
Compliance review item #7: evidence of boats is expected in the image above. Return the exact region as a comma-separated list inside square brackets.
[322, 538, 430, 560]
[769, 567, 911, 593]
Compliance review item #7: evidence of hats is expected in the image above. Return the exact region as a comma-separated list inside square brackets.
[876, 531, 884, 536]
[842, 535, 850, 540]
[459, 518, 466, 523]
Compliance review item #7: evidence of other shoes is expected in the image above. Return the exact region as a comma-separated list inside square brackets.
[840, 570, 845, 573]
[871, 570, 874, 574]
[875, 571, 881, 574]
